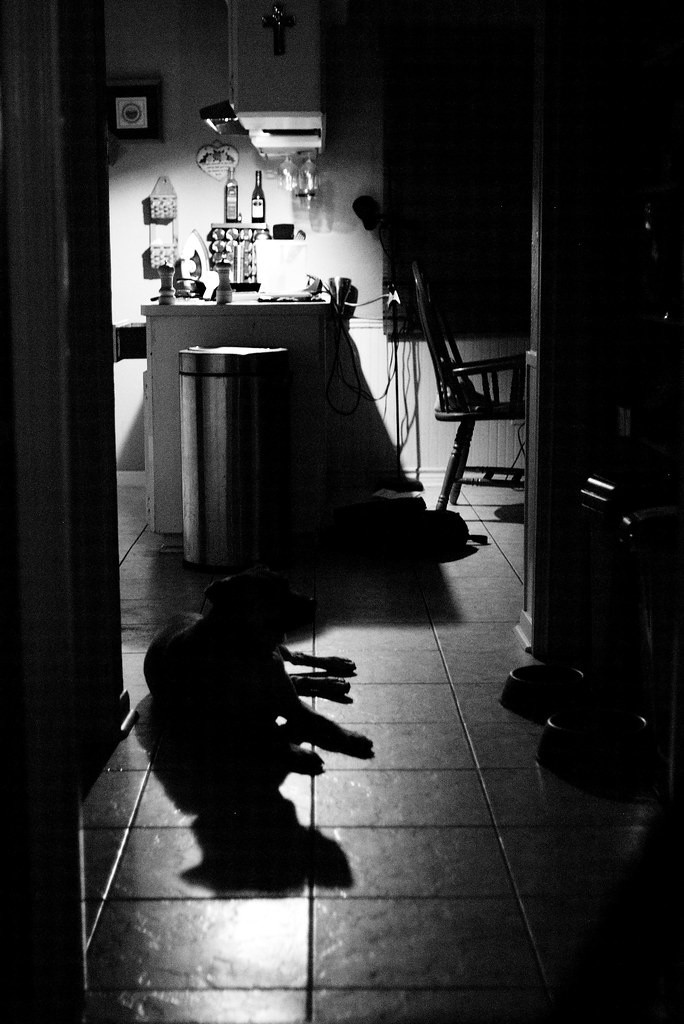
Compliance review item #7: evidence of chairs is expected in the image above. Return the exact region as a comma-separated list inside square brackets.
[413, 261, 525, 513]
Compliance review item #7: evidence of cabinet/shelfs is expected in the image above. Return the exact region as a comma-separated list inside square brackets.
[112, 302, 332, 539]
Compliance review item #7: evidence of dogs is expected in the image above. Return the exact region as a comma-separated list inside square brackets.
[144, 568, 374, 774]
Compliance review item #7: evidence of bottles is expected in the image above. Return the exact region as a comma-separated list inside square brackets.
[216, 260, 233, 303]
[225, 167, 238, 222]
[252, 170, 265, 223]
[158, 260, 175, 305]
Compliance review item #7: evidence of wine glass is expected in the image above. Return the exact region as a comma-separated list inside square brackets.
[265, 152, 319, 190]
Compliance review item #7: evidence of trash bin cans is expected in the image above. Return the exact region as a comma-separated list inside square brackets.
[178, 347, 288, 572]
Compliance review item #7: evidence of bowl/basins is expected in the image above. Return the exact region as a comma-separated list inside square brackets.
[502, 665, 593, 717]
[535, 707, 649, 786]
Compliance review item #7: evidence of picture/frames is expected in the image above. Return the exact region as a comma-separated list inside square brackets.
[107, 79, 165, 143]
